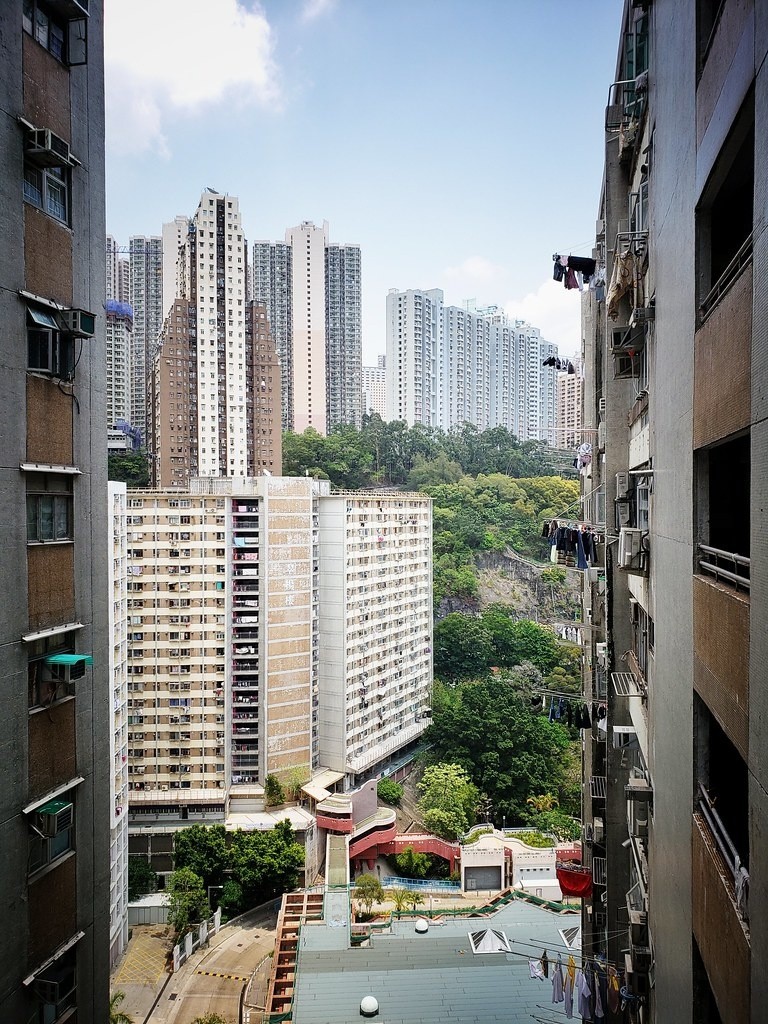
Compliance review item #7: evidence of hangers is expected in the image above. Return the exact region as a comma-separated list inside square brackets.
[541, 690, 608, 708]
[527, 943, 623, 979]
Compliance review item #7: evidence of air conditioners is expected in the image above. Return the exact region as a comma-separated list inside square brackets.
[162, 785, 168, 790]
[35, 800, 73, 838]
[50, 0, 90, 20]
[599, 398, 606, 413]
[26, 127, 70, 168]
[59, 310, 96, 339]
[42, 656, 86, 684]
[617, 527, 641, 569]
[217, 740, 223, 746]
[594, 816, 604, 843]
